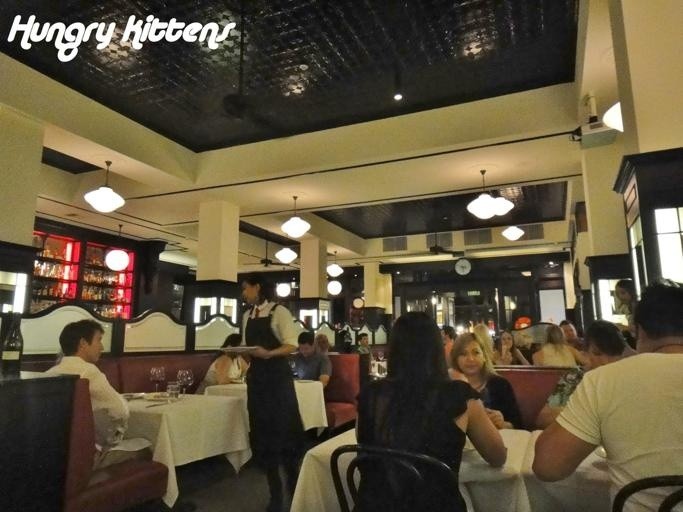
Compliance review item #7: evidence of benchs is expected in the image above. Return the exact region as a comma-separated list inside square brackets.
[494, 365, 579, 431]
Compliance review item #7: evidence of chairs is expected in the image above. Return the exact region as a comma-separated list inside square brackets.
[330, 445, 467, 512]
[611, 475, 683, 511]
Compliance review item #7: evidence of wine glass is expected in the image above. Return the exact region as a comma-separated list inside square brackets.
[288, 359, 296, 370]
[150, 367, 166, 392]
[377, 351, 385, 361]
[177, 369, 194, 399]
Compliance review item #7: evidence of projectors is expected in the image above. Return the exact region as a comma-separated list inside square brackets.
[581, 121, 617, 149]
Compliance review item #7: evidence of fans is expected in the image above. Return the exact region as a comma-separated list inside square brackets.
[242, 240, 289, 268]
[429, 233, 464, 257]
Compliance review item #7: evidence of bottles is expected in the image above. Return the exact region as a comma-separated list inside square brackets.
[468, 318, 495, 333]
[29, 243, 129, 320]
[0, 311, 23, 378]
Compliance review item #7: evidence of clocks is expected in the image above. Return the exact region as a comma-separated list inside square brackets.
[454, 258, 472, 276]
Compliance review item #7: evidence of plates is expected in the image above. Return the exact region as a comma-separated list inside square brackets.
[144, 392, 179, 401]
[230, 379, 243, 384]
[120, 392, 145, 400]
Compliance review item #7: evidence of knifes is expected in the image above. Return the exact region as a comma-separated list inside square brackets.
[146, 403, 168, 408]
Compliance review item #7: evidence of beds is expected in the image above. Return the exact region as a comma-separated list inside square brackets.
[116, 391, 253, 509]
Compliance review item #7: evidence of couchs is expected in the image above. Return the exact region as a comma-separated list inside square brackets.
[1, 349, 359, 512]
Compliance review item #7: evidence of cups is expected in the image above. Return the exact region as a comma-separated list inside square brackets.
[167, 382, 181, 397]
[242, 376, 247, 385]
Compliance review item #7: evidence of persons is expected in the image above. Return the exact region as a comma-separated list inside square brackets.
[293, 331, 333, 389]
[318, 333, 329, 352]
[347, 310, 508, 512]
[194, 333, 250, 396]
[227, 271, 309, 512]
[531, 277, 683, 512]
[44, 317, 154, 472]
[353, 279, 641, 431]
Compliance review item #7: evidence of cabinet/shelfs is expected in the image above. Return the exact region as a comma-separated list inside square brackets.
[30, 216, 167, 320]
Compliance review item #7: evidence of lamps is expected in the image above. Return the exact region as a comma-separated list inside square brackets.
[84, 160, 125, 213]
[281, 196, 311, 238]
[105, 225, 129, 271]
[467, 170, 498, 220]
[326, 251, 344, 277]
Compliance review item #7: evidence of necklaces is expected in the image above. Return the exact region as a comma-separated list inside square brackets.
[651, 343, 683, 353]
